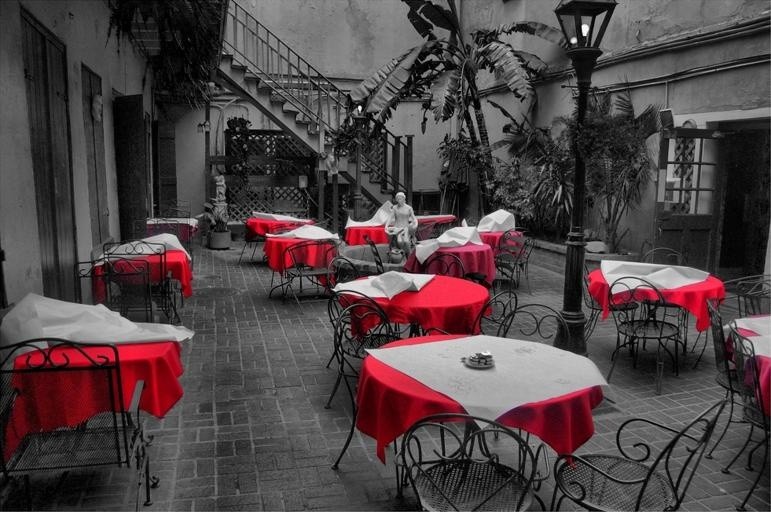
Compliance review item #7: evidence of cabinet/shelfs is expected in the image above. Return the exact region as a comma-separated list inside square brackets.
[480, 349, 494, 366]
[471, 352, 481, 366]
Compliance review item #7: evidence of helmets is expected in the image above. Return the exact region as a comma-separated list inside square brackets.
[463, 357, 497, 369]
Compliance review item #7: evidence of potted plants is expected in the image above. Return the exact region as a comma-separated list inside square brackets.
[194, 202, 238, 249]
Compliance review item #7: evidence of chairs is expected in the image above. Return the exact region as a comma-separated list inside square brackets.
[326, 290, 569, 470]
[239, 200, 535, 312]
[581, 247, 768, 397]
[393, 413, 552, 512]
[705, 300, 769, 511]
[1, 336, 161, 511]
[75, 204, 195, 328]
[551, 398, 730, 510]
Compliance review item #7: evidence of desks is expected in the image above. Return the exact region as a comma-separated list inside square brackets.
[553, 0, 620, 359]
[7, 308, 181, 394]
[354, 336, 607, 490]
[335, 271, 490, 337]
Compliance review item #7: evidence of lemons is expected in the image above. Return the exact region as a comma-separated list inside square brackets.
[384, 248, 409, 264]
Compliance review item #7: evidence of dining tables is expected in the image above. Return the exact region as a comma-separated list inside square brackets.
[194, 202, 238, 249]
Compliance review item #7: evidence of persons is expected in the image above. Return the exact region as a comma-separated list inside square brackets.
[384, 192, 419, 257]
